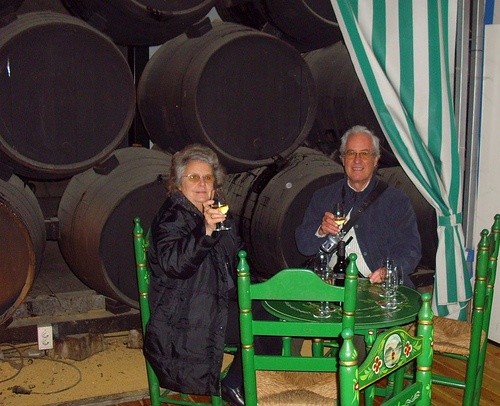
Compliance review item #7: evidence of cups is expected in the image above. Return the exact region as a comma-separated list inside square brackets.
[314, 253, 328, 277]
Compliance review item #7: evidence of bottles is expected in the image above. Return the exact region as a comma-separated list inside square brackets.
[331, 241, 347, 306]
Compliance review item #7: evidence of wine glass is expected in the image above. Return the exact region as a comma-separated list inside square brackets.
[313, 269, 335, 319]
[381, 266, 399, 309]
[379, 257, 394, 297]
[332, 203, 344, 240]
[209, 187, 231, 232]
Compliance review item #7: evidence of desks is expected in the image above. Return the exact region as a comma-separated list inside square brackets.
[261, 276, 423, 406]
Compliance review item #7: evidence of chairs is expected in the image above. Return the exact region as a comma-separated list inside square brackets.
[134, 214, 500, 406]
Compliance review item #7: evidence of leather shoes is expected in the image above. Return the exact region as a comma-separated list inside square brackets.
[220, 377, 246, 406]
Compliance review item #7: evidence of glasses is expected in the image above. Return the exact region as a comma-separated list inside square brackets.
[181, 174, 216, 183]
[344, 151, 376, 160]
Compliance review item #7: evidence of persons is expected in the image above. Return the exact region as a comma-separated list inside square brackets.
[295, 124, 425, 364]
[145, 145, 254, 404]
